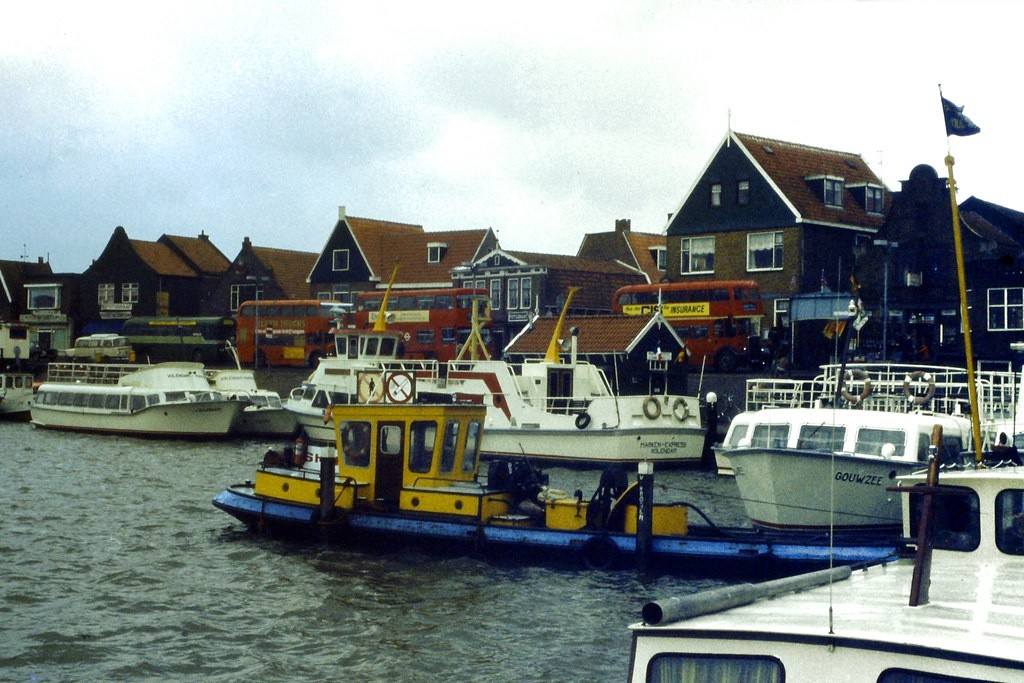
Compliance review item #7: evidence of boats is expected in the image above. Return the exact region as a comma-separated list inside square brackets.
[0, 81, 1024, 683]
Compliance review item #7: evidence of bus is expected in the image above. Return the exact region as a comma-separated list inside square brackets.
[119, 317, 236, 367]
[236, 300, 350, 369]
[354, 287, 494, 361]
[610, 281, 765, 376]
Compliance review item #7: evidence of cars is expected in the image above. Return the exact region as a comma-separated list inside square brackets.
[29, 340, 58, 363]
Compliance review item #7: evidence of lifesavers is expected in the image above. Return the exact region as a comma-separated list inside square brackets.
[575, 413, 591, 430]
[579, 534, 620, 572]
[840, 368, 872, 401]
[644, 396, 662, 420]
[902, 370, 935, 402]
[673, 397, 690, 422]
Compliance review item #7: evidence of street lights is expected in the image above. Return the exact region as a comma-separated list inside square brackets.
[873, 238, 900, 363]
[461, 261, 488, 297]
[246, 274, 271, 369]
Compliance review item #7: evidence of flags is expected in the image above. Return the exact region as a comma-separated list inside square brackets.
[940, 92, 980, 137]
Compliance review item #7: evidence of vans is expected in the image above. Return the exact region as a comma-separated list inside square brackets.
[74, 334, 136, 363]
